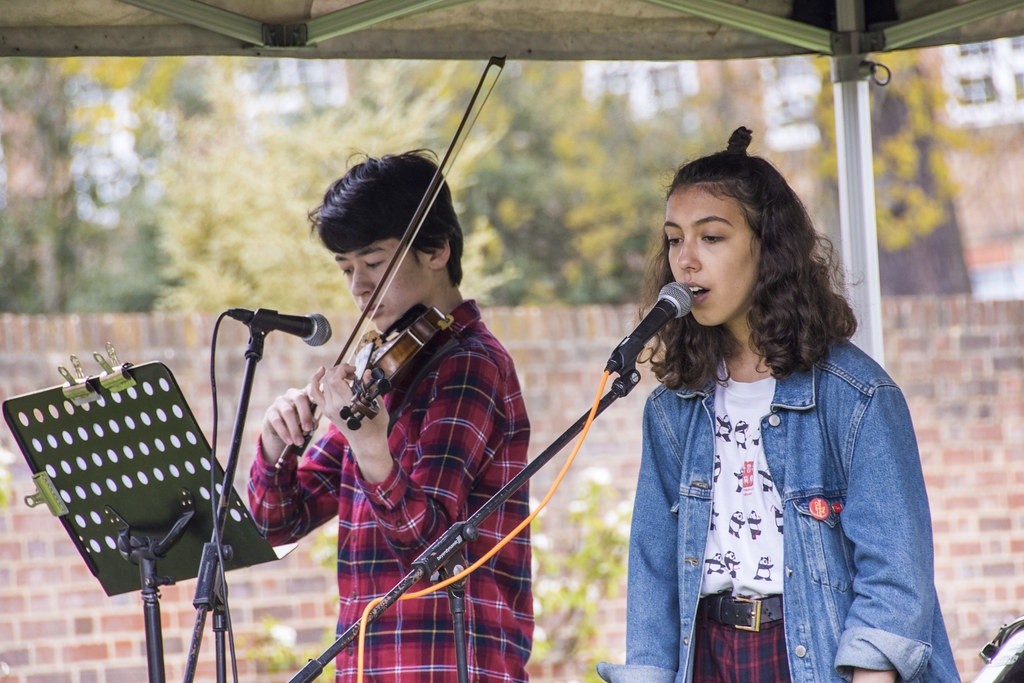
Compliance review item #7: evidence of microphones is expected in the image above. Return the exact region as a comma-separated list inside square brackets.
[226, 307, 332, 346]
[604, 281, 694, 375]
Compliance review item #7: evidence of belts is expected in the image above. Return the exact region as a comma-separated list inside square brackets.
[696, 594, 783, 631]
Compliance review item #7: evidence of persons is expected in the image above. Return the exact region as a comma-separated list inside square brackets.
[596, 125, 964, 683]
[247, 149, 533, 683]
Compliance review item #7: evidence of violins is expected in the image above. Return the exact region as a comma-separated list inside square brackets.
[337, 305, 456, 432]
